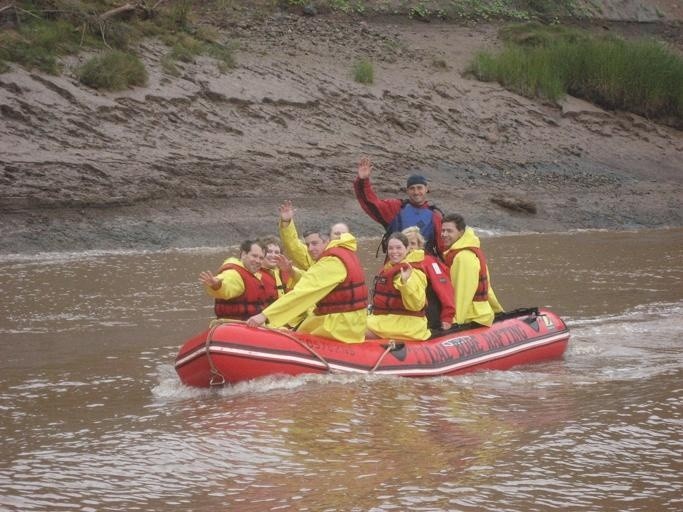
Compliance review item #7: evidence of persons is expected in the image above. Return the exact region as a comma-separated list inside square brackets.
[199, 200, 369, 344]
[354, 158, 504, 341]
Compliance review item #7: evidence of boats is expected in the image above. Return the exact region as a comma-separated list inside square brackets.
[174, 305, 570, 390]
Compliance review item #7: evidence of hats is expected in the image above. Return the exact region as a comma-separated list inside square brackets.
[407, 175, 427, 188]
